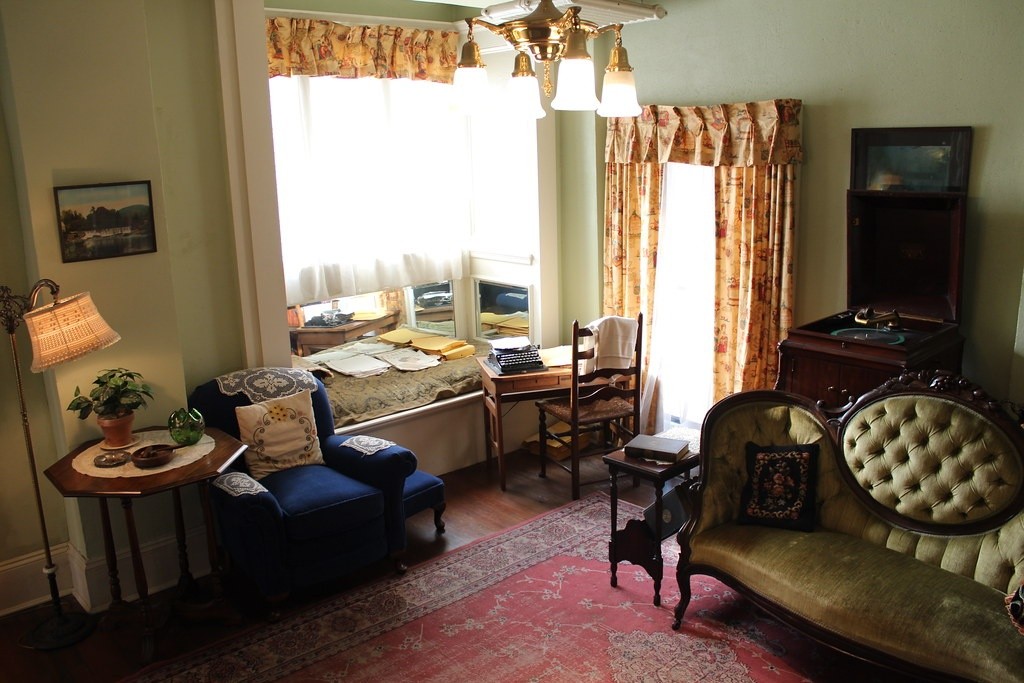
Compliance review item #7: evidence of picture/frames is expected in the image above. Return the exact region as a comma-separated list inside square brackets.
[53, 180, 157, 263]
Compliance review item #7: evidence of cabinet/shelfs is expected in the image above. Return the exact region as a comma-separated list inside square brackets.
[774, 125, 974, 407]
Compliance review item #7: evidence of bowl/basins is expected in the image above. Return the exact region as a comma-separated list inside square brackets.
[131, 443, 175, 467]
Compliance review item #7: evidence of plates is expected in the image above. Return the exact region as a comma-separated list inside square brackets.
[93, 450, 131, 466]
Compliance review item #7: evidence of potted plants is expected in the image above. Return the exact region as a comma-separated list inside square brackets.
[67, 369, 155, 451]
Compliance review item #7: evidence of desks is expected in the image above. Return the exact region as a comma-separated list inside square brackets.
[295, 310, 399, 356]
[602, 422, 705, 607]
[476, 349, 632, 491]
[42, 425, 248, 637]
[414, 304, 455, 322]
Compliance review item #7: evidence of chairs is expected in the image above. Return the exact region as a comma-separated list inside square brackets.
[535, 313, 644, 500]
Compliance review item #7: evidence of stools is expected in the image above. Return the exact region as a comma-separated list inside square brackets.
[402, 470, 447, 530]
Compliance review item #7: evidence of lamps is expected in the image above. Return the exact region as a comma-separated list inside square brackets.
[0, 279, 123, 652]
[458, 0, 643, 120]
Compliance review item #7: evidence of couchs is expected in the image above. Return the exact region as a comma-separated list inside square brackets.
[190, 366, 418, 616]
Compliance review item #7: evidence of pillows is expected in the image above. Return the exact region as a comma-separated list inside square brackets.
[1004, 583, 1024, 635]
[232, 390, 326, 480]
[735, 441, 816, 532]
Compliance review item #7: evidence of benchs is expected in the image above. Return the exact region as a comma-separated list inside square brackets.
[672, 370, 1024, 683]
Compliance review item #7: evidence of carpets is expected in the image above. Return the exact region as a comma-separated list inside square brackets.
[110, 493, 926, 683]
